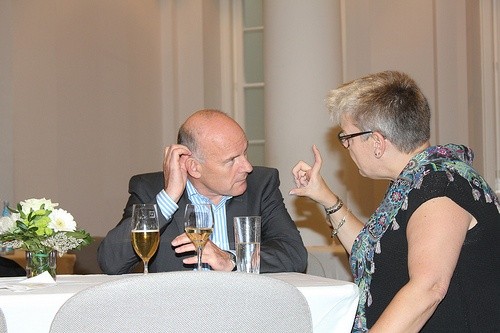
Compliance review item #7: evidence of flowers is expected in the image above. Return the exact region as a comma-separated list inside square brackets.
[0, 196, 94, 254]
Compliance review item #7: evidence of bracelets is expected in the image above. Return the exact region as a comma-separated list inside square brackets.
[325, 197, 344, 214]
[330, 209, 352, 238]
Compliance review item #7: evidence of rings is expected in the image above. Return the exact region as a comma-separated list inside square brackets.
[302, 173, 306, 177]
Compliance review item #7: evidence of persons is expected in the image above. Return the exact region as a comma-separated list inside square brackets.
[97, 109, 308, 276]
[287, 70, 500, 333]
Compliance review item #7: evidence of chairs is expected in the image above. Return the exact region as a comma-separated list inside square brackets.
[48, 270, 312, 333]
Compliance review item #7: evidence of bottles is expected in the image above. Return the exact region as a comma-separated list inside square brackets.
[1, 201, 14, 255]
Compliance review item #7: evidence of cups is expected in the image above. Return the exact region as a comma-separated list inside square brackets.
[233, 215, 261, 274]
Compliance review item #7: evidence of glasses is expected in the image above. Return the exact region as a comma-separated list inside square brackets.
[338, 131, 386, 150]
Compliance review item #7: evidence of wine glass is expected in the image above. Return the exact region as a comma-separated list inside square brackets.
[131, 204, 160, 274]
[183, 203, 213, 271]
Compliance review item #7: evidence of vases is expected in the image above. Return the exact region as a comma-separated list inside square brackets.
[25, 250, 58, 282]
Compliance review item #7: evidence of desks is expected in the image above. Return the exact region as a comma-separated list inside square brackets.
[1, 269, 360, 333]
